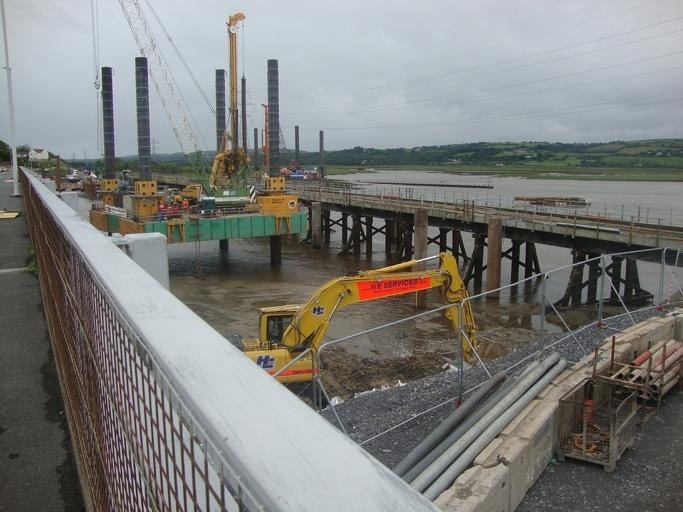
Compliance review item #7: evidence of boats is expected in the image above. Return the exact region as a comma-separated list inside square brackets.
[528, 193, 592, 209]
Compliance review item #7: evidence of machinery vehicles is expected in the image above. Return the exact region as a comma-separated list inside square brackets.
[237, 244, 490, 385]
